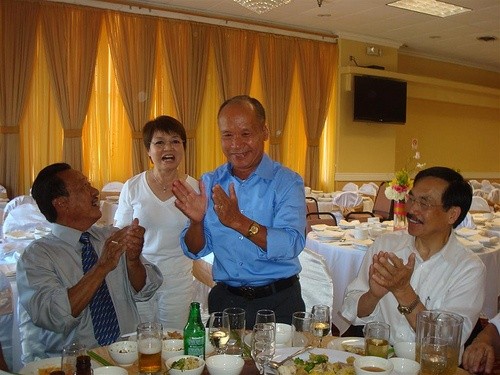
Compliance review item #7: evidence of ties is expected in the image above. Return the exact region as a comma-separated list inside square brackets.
[79, 232, 121, 346]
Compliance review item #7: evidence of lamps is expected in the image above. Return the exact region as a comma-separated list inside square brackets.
[366, 46, 384, 56]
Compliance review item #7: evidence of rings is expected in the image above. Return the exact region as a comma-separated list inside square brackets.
[219, 206, 221, 208]
[112, 241, 118, 244]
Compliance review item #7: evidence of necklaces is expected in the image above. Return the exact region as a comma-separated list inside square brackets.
[152, 171, 174, 192]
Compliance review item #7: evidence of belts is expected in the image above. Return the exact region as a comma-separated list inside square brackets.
[227, 275, 298, 300]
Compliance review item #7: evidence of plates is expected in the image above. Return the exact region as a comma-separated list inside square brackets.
[311, 220, 500, 252]
[18, 328, 395, 375]
[317, 197, 370, 202]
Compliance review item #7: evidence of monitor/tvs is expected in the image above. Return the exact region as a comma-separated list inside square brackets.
[353, 76, 407, 123]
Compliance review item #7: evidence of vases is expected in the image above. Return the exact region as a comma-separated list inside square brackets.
[392, 199, 407, 230]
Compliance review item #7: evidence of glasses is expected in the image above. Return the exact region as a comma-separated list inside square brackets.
[404, 192, 448, 209]
[148, 138, 185, 146]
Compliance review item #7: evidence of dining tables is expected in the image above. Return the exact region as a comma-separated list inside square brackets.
[19, 332, 471, 375]
[98, 200, 119, 226]
[0, 229, 51, 369]
[309, 221, 500, 331]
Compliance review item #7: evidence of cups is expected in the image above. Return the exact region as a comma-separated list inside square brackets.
[223, 308, 246, 357]
[304, 187, 363, 199]
[63, 343, 86, 375]
[354, 217, 389, 241]
[291, 311, 315, 347]
[414, 310, 464, 375]
[363, 322, 390, 359]
[137, 321, 163, 375]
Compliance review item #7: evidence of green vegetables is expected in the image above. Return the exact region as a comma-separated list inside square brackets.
[164, 359, 184, 375]
[292, 355, 329, 372]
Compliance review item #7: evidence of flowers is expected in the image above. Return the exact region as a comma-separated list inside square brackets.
[384, 152, 426, 201]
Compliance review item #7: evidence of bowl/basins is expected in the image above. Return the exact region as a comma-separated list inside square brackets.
[206, 355, 245, 375]
[353, 342, 420, 375]
[107, 340, 139, 366]
[93, 366, 129, 375]
[164, 354, 206, 375]
[161, 339, 184, 361]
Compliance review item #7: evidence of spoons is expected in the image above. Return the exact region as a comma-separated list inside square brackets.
[266, 345, 313, 369]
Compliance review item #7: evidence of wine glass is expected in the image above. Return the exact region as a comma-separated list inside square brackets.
[207, 304, 331, 375]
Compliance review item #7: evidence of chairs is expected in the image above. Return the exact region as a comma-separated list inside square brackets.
[0, 183, 47, 236]
[301, 180, 500, 229]
[100, 180, 124, 199]
[298, 248, 334, 335]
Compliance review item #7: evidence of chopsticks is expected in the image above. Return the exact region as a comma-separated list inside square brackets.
[86, 349, 114, 366]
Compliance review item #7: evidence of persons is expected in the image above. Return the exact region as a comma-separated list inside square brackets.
[113, 115, 201, 331]
[462, 312, 500, 373]
[339, 167, 487, 366]
[16, 162, 164, 366]
[172, 95, 308, 331]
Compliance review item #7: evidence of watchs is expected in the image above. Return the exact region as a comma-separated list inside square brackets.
[397, 295, 420, 315]
[246, 222, 259, 238]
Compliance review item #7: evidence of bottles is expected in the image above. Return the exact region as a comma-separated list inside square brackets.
[183, 301, 205, 361]
[76, 355, 91, 375]
